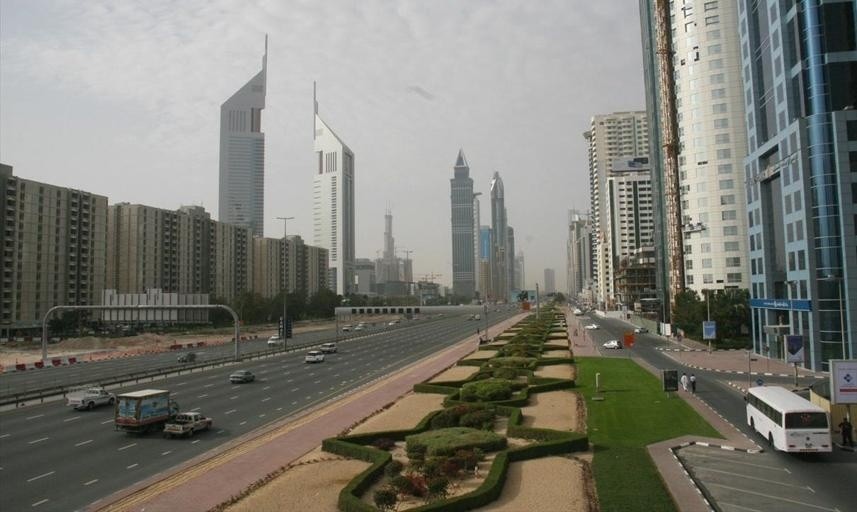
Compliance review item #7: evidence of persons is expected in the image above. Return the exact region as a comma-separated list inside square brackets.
[839, 416, 854, 446]
[690, 373, 696, 395]
[680, 373, 689, 392]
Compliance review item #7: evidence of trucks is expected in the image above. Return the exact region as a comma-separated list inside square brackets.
[113, 388, 181, 437]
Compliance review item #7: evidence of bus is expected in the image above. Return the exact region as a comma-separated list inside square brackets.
[741, 385, 836, 459]
[585, 323, 601, 330]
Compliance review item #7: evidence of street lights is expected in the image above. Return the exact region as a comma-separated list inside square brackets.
[274, 216, 296, 348]
[825, 272, 847, 360]
[658, 286, 666, 337]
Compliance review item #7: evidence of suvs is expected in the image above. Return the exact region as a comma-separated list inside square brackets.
[304, 350, 326, 364]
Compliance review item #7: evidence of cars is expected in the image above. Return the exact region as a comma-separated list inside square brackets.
[475, 313, 481, 321]
[634, 326, 649, 334]
[229, 369, 255, 384]
[342, 320, 373, 333]
[176, 351, 195, 364]
[388, 320, 401, 326]
[319, 342, 338, 353]
[266, 334, 285, 347]
[567, 299, 590, 315]
[488, 301, 522, 313]
[601, 339, 622, 350]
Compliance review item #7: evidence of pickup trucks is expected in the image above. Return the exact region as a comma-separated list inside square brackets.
[65, 386, 116, 412]
[161, 411, 213, 439]
